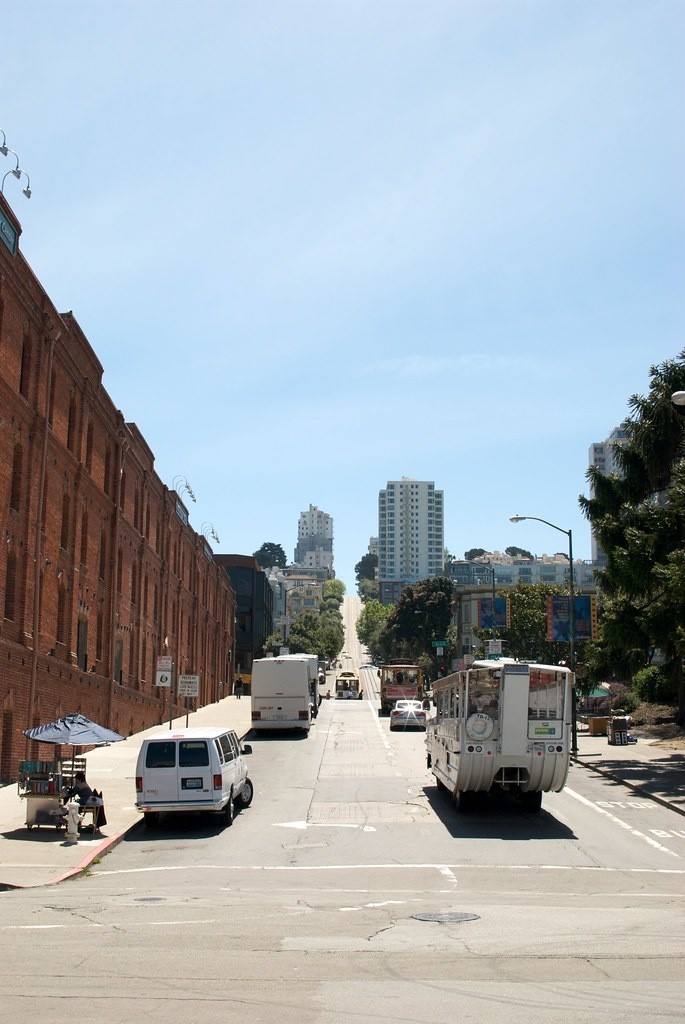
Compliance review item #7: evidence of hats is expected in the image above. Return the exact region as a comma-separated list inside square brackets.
[73, 773, 84, 781]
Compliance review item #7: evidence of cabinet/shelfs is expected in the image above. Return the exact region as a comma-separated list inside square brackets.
[18, 756, 87, 795]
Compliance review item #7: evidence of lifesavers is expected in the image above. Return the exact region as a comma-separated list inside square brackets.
[465, 712, 493, 742]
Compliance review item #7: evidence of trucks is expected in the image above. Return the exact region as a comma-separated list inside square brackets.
[277, 653, 322, 719]
[425, 658, 576, 813]
[251, 657, 314, 733]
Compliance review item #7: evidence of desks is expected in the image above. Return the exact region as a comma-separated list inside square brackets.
[20, 793, 61, 832]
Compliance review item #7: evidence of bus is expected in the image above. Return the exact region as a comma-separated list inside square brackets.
[377, 665, 425, 716]
[334, 672, 360, 700]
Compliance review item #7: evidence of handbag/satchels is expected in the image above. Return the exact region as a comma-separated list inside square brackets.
[88, 796, 103, 807]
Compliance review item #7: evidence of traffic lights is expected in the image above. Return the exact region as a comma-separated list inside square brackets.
[439, 666, 445, 671]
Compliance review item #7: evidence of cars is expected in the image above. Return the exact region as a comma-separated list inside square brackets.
[390, 700, 426, 731]
[342, 651, 350, 657]
[318, 657, 331, 683]
[423, 692, 430, 711]
[372, 652, 414, 667]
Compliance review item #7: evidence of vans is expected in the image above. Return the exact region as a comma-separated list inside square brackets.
[134, 727, 254, 826]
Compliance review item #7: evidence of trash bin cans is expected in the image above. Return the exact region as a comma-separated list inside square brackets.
[606, 716, 628, 746]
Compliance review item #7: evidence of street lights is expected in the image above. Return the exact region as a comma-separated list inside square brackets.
[284, 582, 318, 647]
[415, 609, 443, 679]
[452, 558, 502, 660]
[510, 514, 578, 757]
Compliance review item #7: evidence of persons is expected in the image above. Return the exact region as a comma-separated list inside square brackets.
[326, 690, 330, 700]
[21, 711, 126, 803]
[450, 699, 499, 720]
[235, 676, 243, 699]
[359, 689, 363, 700]
[63, 772, 92, 832]
[396, 669, 403, 685]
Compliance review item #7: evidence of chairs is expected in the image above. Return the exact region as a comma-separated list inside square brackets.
[79, 789, 102, 837]
